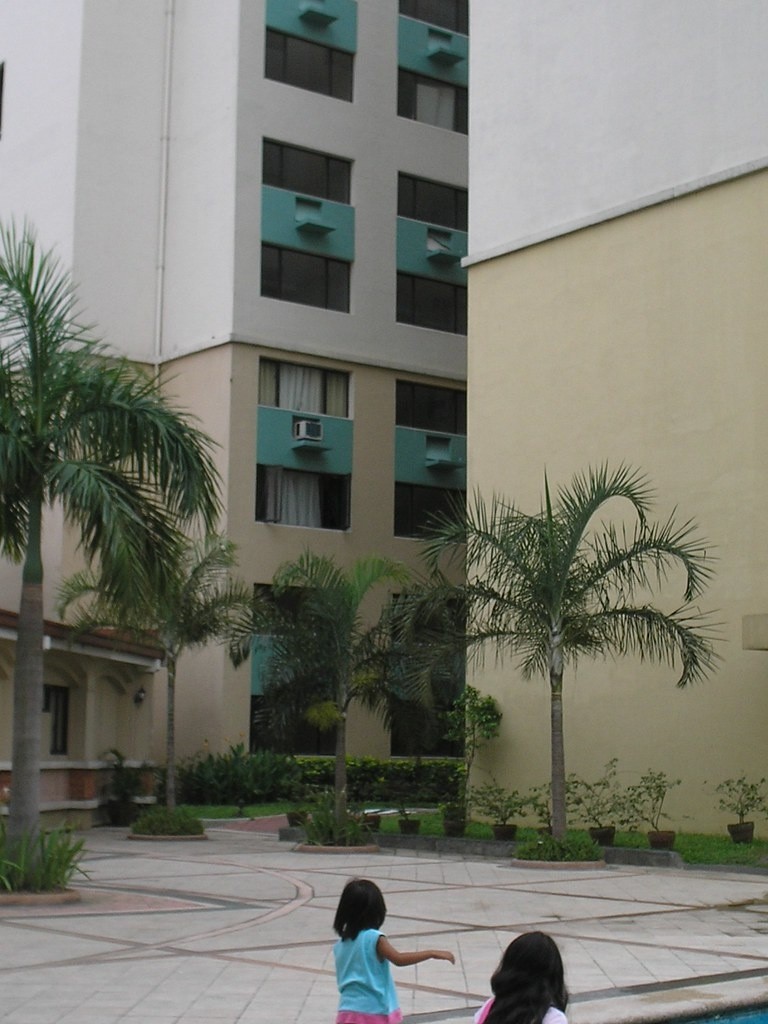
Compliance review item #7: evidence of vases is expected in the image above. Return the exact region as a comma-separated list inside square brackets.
[357, 813, 381, 832]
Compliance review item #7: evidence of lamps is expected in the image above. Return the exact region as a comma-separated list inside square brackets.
[134, 684, 147, 704]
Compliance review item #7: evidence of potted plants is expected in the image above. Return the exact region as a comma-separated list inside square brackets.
[270, 797, 308, 828]
[564, 761, 622, 846]
[437, 684, 504, 820]
[304, 814, 332, 847]
[333, 815, 363, 846]
[438, 801, 473, 838]
[701, 768, 768, 843]
[397, 798, 421, 834]
[610, 767, 695, 851]
[101, 746, 150, 826]
[468, 770, 541, 841]
[528, 781, 581, 836]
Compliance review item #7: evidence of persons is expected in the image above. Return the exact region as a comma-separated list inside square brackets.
[474, 930, 572, 1024]
[332, 878, 455, 1024]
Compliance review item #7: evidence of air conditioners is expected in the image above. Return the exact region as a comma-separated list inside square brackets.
[294, 420, 323, 441]
